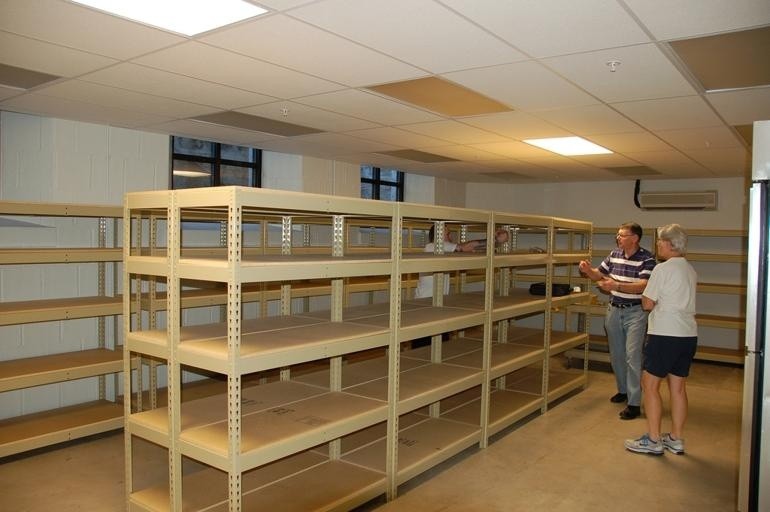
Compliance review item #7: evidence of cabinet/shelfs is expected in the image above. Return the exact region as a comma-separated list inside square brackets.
[1, 199, 141, 456]
[122, 185, 592, 511]
[584, 225, 749, 364]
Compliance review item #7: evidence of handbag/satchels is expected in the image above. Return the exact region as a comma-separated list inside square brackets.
[528, 283, 574, 297]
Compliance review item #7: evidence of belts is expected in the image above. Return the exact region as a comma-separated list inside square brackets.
[609, 300, 642, 309]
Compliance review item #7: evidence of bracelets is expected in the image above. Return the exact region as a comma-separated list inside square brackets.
[615, 282, 621, 291]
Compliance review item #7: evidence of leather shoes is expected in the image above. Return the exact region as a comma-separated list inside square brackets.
[610, 393, 627, 403]
[618, 405, 640, 419]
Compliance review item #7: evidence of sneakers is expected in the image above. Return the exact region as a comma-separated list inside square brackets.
[662, 432, 685, 455]
[623, 433, 665, 456]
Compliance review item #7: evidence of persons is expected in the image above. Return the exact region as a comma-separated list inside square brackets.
[410, 225, 508, 349]
[624, 227, 697, 454]
[578, 223, 656, 419]
